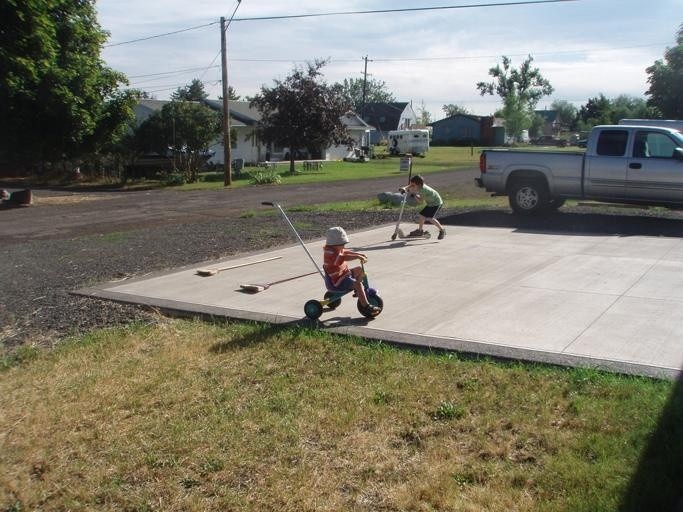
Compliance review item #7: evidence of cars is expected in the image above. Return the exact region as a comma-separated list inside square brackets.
[529, 134, 570, 149]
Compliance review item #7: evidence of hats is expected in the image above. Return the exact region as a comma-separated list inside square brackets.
[326, 227, 349, 246]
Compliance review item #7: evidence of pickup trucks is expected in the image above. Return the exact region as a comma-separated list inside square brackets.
[475, 122, 683, 217]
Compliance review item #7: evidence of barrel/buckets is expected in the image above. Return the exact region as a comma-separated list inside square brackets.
[399, 152, 413, 171]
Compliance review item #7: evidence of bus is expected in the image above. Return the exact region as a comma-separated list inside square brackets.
[386, 128, 430, 158]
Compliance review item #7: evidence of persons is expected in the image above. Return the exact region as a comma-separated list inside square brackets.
[404, 175, 446, 240]
[323, 226, 382, 316]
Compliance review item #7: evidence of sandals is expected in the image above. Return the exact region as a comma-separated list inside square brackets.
[363, 304, 381, 312]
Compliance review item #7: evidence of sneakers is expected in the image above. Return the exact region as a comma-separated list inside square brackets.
[438, 229, 446, 239]
[411, 229, 423, 236]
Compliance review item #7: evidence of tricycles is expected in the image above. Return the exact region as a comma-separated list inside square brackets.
[259, 199, 385, 320]
